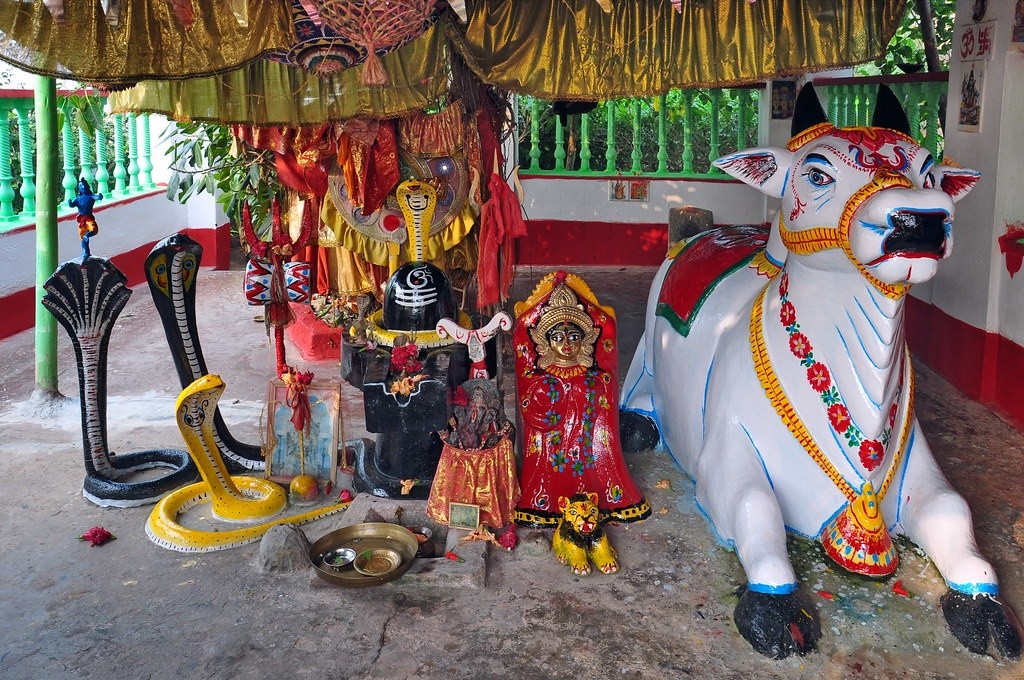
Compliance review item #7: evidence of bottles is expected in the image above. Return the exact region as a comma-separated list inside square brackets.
[407, 526, 435, 559]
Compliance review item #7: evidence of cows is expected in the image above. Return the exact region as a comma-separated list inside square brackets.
[619, 82, 1024, 661]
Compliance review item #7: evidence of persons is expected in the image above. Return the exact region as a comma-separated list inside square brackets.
[68, 176, 104, 259]
[511, 270, 653, 532]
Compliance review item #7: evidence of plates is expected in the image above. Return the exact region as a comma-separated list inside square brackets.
[354, 547, 401, 576]
[322, 546, 356, 570]
[309, 522, 419, 586]
